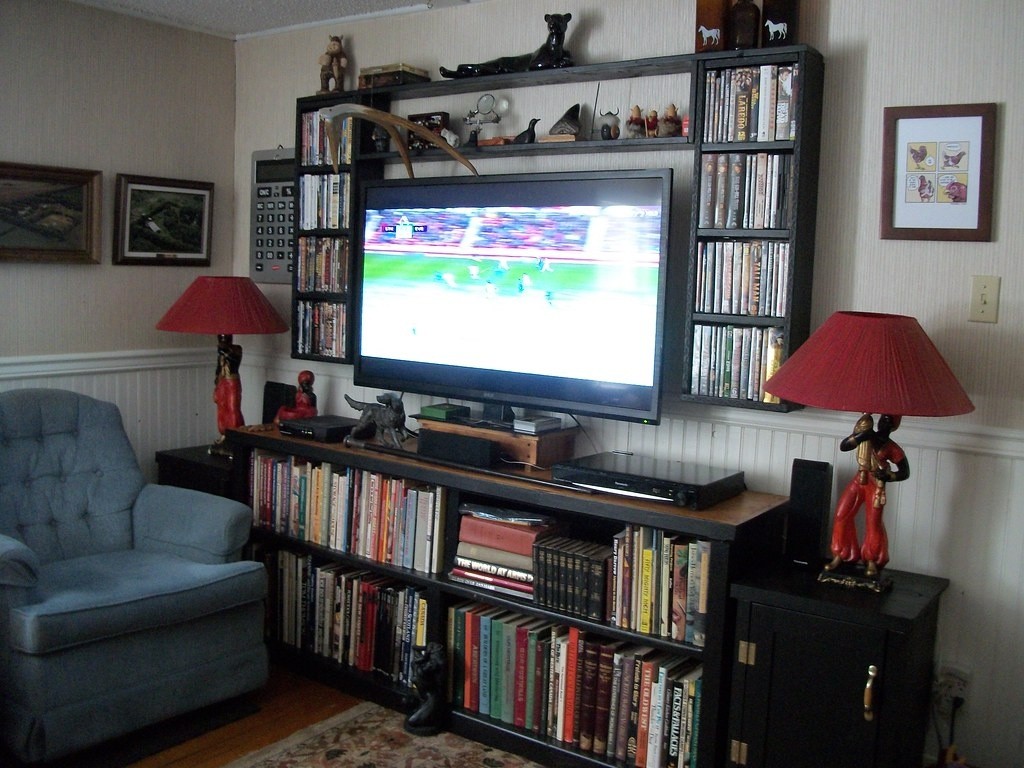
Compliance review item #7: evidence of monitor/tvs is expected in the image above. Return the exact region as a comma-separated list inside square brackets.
[353, 168, 675, 432]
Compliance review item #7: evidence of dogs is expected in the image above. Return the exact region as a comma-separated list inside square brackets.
[344, 393, 408, 449]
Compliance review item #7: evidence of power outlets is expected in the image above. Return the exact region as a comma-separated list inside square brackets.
[937, 664, 978, 717]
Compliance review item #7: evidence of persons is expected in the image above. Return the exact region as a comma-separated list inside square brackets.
[825, 410, 910, 577]
[273, 370, 319, 426]
[213, 334, 245, 446]
[625, 103, 681, 138]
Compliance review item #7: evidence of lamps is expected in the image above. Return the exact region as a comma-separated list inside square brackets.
[155, 275, 287, 462]
[764, 312, 975, 594]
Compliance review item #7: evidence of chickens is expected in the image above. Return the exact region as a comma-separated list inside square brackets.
[918, 175, 933, 202]
[910, 146, 927, 169]
[940, 151, 966, 169]
[944, 182, 967, 202]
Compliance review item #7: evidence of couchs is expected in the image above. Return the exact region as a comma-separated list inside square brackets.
[0, 386, 272, 764]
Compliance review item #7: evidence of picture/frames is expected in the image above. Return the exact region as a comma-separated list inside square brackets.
[880, 102, 996, 241]
[0, 161, 103, 265]
[114, 173, 215, 268]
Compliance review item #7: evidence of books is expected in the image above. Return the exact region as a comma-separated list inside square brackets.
[690, 62, 799, 405]
[242, 449, 711, 768]
[296, 111, 353, 358]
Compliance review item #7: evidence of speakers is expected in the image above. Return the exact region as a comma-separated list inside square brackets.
[262, 381, 297, 425]
[417, 428, 504, 469]
[782, 458, 833, 573]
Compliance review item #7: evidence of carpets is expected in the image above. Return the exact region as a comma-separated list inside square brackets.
[216, 700, 552, 768]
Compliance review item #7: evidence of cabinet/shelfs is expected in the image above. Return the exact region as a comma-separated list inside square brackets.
[289, 45, 823, 414]
[725, 552, 950, 768]
[156, 444, 244, 498]
[226, 421, 790, 768]
